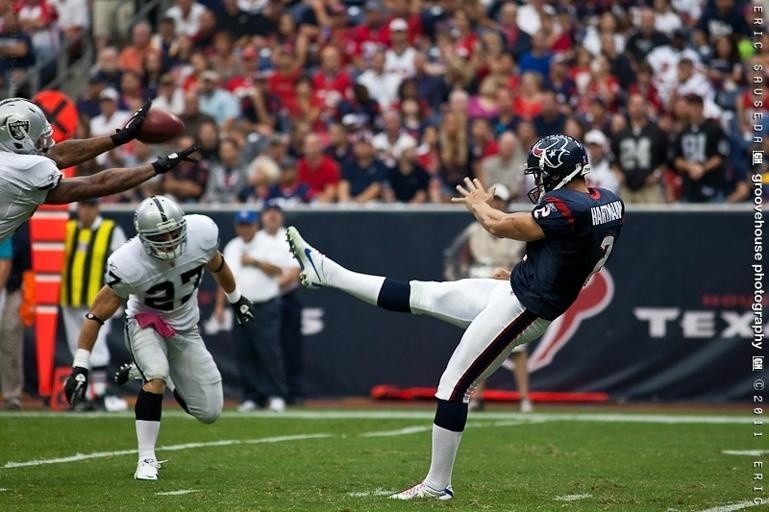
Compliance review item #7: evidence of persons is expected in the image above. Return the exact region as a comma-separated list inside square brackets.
[0, 93, 210, 244]
[1, 219, 34, 410]
[63, 192, 257, 483]
[440, 181, 536, 416]
[281, 132, 627, 503]
[255, 204, 308, 406]
[212, 207, 290, 414]
[2, 1, 767, 212]
[57, 197, 129, 413]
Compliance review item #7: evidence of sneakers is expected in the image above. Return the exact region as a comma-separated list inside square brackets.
[284, 226, 327, 289]
[520, 400, 533, 412]
[115, 360, 144, 385]
[238, 400, 255, 412]
[389, 481, 453, 501]
[468, 398, 480, 411]
[270, 396, 284, 411]
[135, 456, 167, 480]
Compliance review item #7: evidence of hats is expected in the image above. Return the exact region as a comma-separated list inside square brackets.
[488, 183, 511, 201]
[234, 209, 258, 223]
[261, 197, 282, 210]
[74, 198, 101, 207]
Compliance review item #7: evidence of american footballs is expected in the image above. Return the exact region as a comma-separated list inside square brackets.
[140, 108, 184, 143]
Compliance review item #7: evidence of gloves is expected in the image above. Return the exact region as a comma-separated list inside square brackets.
[63, 347, 91, 406]
[153, 142, 202, 173]
[226, 289, 254, 327]
[109, 97, 152, 146]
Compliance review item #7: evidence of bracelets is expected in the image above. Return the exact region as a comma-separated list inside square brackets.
[223, 283, 242, 305]
[71, 347, 90, 368]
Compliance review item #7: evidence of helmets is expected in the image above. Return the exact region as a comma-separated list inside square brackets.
[136, 196, 188, 260]
[524, 134, 590, 205]
[0, 97, 55, 158]
